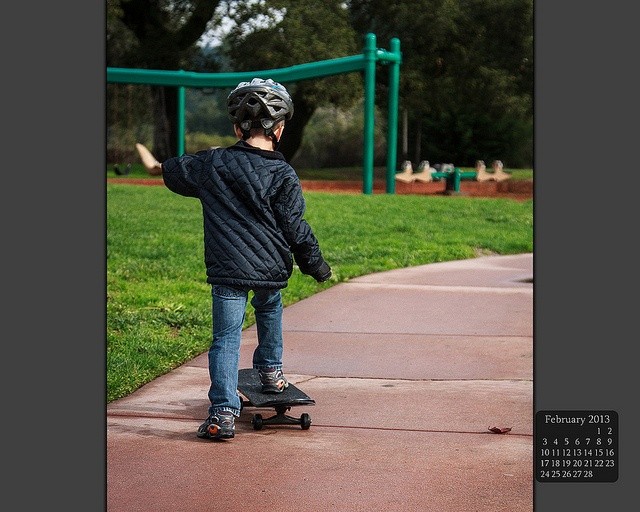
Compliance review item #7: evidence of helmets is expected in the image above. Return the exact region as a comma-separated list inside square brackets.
[225, 77, 295, 130]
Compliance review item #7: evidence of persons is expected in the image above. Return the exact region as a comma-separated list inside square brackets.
[135, 77, 333, 440]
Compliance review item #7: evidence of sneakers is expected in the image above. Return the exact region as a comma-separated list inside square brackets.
[257, 370, 289, 393]
[197, 411, 236, 439]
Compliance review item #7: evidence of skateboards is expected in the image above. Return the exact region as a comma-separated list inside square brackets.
[235, 368, 316, 431]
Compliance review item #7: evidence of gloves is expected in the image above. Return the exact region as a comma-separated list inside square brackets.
[310, 260, 332, 282]
